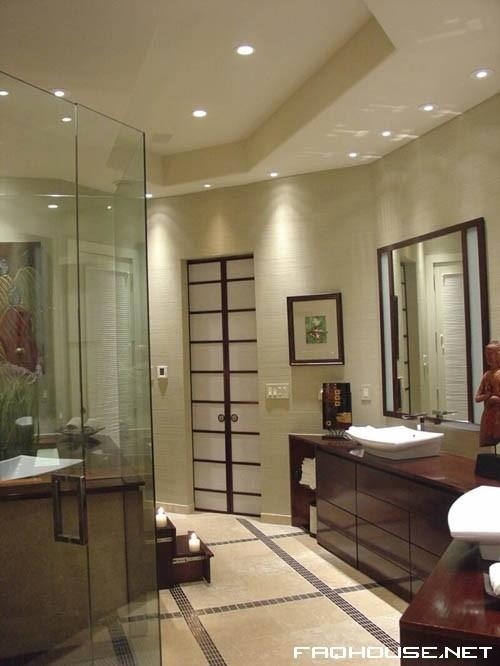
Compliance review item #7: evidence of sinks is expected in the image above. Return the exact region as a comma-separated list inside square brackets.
[447, 486, 500, 559]
[344, 425, 444, 457]
[0, 448, 85, 489]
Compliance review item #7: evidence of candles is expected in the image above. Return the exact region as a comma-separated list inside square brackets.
[189, 533, 200, 552]
[156, 507, 167, 528]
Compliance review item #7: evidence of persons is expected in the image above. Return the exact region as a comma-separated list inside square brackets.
[473, 341, 500, 448]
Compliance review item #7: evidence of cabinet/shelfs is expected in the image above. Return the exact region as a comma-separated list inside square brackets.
[409, 479, 461, 605]
[356, 462, 410, 605]
[315, 446, 357, 567]
[288, 434, 318, 540]
[400, 539, 499, 665]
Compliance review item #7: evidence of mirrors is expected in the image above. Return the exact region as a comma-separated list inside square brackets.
[376, 217, 489, 425]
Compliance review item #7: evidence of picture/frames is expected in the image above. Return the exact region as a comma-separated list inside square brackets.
[286, 293, 344, 367]
[0, 238, 59, 423]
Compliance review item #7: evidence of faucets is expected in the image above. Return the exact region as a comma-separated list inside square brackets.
[403, 410, 428, 432]
[431, 409, 458, 425]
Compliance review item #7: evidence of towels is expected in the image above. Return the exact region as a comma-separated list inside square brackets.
[299, 458, 316, 489]
[66, 417, 84, 431]
[485, 561, 500, 595]
[83, 417, 98, 434]
